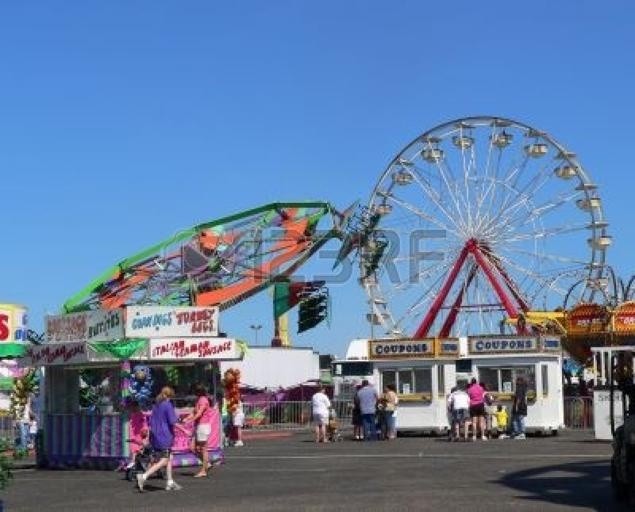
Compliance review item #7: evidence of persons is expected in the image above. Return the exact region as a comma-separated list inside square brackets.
[310, 385, 333, 444]
[356, 378, 380, 442]
[445, 377, 530, 443]
[132, 384, 193, 494]
[175, 384, 213, 481]
[229, 393, 247, 448]
[383, 384, 400, 442]
[350, 382, 364, 441]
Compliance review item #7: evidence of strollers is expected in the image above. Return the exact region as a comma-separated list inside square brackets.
[312, 409, 343, 444]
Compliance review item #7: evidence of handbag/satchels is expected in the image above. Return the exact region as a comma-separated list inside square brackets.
[197, 424, 211, 442]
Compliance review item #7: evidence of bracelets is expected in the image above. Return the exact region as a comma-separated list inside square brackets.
[177, 417, 185, 422]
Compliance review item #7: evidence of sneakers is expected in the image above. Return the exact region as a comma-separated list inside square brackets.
[165, 482, 181, 491]
[136, 474, 144, 493]
[234, 441, 243, 447]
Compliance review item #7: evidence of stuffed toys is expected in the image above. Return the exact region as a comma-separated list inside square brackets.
[134, 365, 153, 409]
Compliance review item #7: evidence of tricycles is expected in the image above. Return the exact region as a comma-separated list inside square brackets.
[123, 438, 165, 481]
